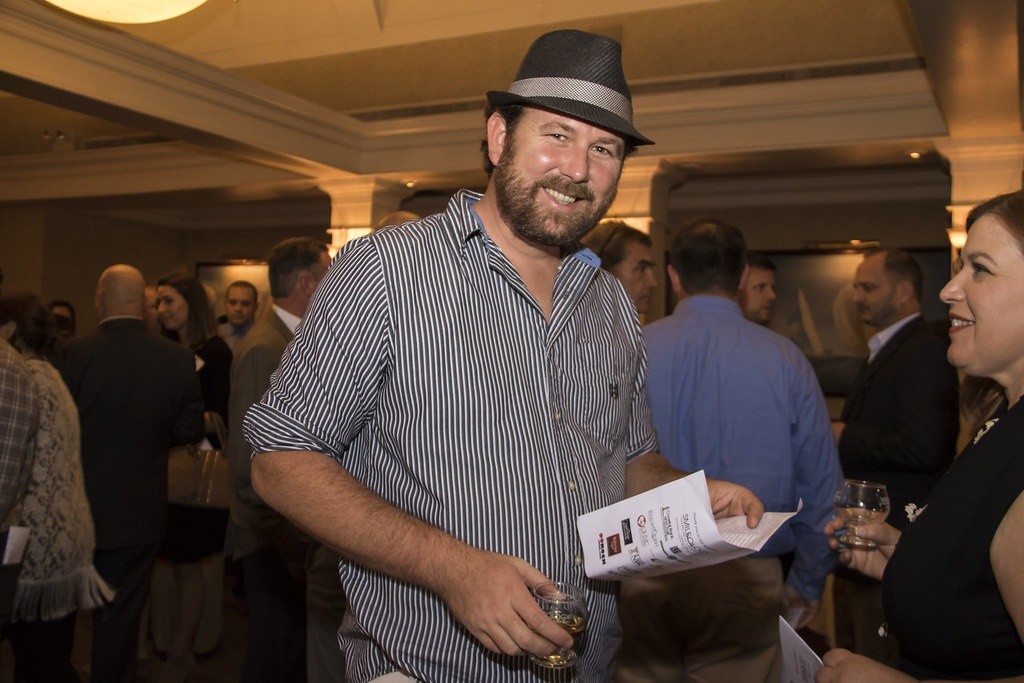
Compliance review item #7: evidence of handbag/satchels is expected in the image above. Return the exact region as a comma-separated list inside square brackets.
[166, 413, 232, 511]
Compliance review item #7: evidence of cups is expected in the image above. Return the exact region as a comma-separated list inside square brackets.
[530, 580, 588, 668]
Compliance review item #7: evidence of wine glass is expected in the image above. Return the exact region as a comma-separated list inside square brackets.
[835, 480, 892, 548]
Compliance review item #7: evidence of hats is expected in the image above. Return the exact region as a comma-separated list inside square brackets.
[486, 29, 655, 147]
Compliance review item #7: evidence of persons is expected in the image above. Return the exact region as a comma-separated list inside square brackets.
[831, 246, 957, 667]
[0, 263, 233, 683]
[613, 219, 846, 683]
[242, 30, 765, 683]
[813, 190, 1024, 682]
[740, 249, 776, 326]
[580, 220, 657, 314]
[217, 238, 332, 683]
